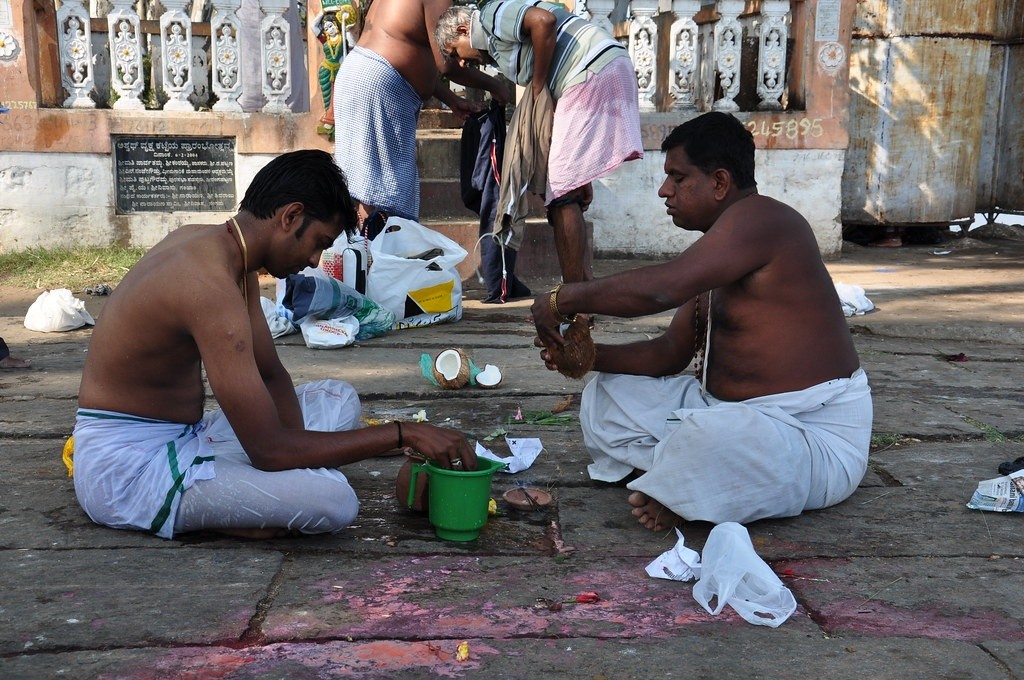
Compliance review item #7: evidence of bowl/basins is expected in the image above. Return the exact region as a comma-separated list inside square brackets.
[503, 488, 553, 510]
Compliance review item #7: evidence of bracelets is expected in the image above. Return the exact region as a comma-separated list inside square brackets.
[394, 420, 403, 449]
[550, 283, 579, 324]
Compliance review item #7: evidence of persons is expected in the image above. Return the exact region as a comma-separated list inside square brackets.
[436, 0, 643, 330]
[530, 111, 873, 532]
[74, 149, 477, 537]
[333, 0, 510, 232]
[310, 6, 357, 130]
[868, 226, 941, 247]
[0, 337, 32, 368]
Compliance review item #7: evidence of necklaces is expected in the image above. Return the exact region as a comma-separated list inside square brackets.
[226, 216, 249, 307]
[694, 193, 758, 379]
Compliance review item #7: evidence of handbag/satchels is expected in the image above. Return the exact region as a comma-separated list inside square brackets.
[24, 288, 95, 332]
[692, 522, 797, 628]
[277, 213, 468, 350]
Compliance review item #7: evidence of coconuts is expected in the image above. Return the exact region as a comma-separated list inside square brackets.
[544, 322, 595, 381]
[432, 349, 502, 388]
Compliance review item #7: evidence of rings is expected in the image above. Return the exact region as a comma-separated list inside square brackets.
[450, 457, 463, 466]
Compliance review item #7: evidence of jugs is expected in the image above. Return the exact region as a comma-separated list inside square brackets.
[407, 454, 507, 542]
